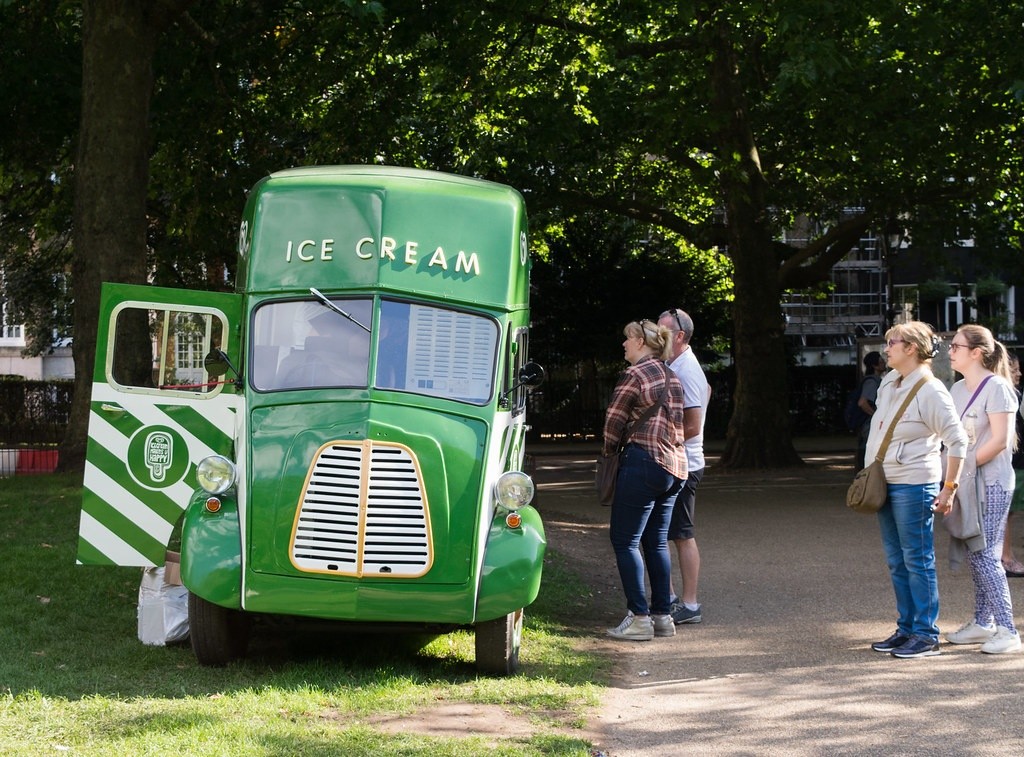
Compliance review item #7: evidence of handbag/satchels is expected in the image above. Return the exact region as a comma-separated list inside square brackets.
[595, 451, 619, 506]
[846, 460, 888, 515]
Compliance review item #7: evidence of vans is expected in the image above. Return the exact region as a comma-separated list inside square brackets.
[75, 164, 549, 675]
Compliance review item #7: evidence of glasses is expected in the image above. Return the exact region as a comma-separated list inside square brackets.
[948, 343, 973, 351]
[638, 319, 646, 345]
[670, 310, 683, 331]
[889, 339, 911, 348]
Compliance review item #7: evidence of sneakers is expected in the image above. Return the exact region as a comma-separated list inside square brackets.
[606, 610, 654, 640]
[942, 619, 997, 644]
[891, 633, 941, 658]
[872, 629, 910, 652]
[981, 626, 1022, 654]
[647, 595, 679, 615]
[649, 614, 675, 636]
[669, 601, 702, 624]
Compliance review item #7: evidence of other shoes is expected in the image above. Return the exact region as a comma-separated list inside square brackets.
[1002, 556, 1024, 577]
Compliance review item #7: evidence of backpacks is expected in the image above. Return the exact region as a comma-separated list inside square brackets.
[843, 377, 876, 433]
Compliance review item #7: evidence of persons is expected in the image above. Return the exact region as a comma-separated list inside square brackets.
[602, 320, 689, 640]
[654, 309, 712, 623]
[854, 351, 887, 469]
[865, 322, 969, 658]
[942, 325, 1024, 653]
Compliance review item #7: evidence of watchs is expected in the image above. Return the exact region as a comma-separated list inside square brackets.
[944, 481, 960, 490]
